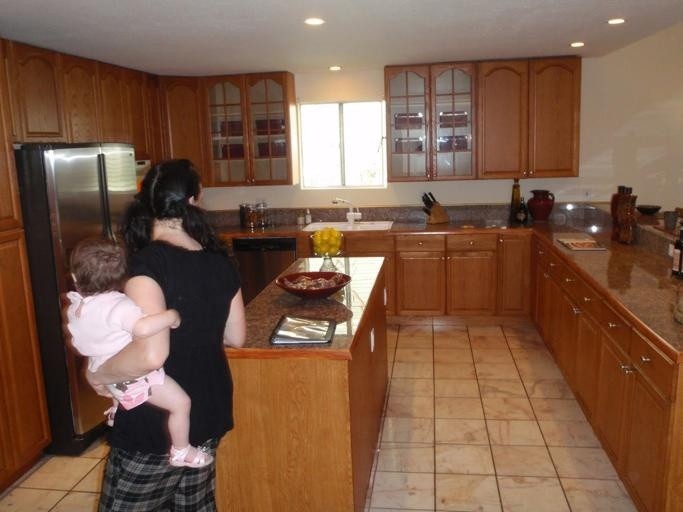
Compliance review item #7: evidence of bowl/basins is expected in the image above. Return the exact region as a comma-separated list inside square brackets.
[636, 205, 661, 216]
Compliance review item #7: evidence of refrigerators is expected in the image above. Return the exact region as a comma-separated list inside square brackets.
[13, 139, 136, 456]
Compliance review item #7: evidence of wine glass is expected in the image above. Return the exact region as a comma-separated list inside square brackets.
[310, 234, 343, 271]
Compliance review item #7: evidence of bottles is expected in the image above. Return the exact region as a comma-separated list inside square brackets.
[511, 176, 528, 225]
[669, 217, 683, 275]
[296, 209, 311, 225]
[238, 199, 267, 230]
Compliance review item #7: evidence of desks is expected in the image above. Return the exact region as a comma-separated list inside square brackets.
[215, 257, 386, 511]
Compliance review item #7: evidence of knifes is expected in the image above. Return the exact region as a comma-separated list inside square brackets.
[421, 191, 437, 216]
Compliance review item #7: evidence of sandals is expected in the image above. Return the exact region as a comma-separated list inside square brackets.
[103, 406, 118, 427]
[169, 445, 213, 469]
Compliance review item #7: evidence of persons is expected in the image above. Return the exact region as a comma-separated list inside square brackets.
[63, 235, 214, 469]
[96, 158, 247, 511]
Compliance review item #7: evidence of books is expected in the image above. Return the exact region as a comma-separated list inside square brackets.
[269, 313, 334, 345]
[555, 237, 607, 251]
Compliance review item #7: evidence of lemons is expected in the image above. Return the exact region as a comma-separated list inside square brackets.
[313, 227, 341, 257]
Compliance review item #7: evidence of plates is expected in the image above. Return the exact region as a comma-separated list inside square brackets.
[275, 270, 351, 298]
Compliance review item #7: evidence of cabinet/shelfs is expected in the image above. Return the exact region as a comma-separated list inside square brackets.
[0, 229, 51, 489]
[395, 234, 500, 318]
[385, 62, 477, 181]
[4, 39, 164, 166]
[158, 73, 213, 189]
[477, 57, 580, 180]
[549, 262, 604, 424]
[201, 70, 300, 187]
[499, 231, 533, 318]
[534, 237, 564, 349]
[589, 300, 681, 511]
